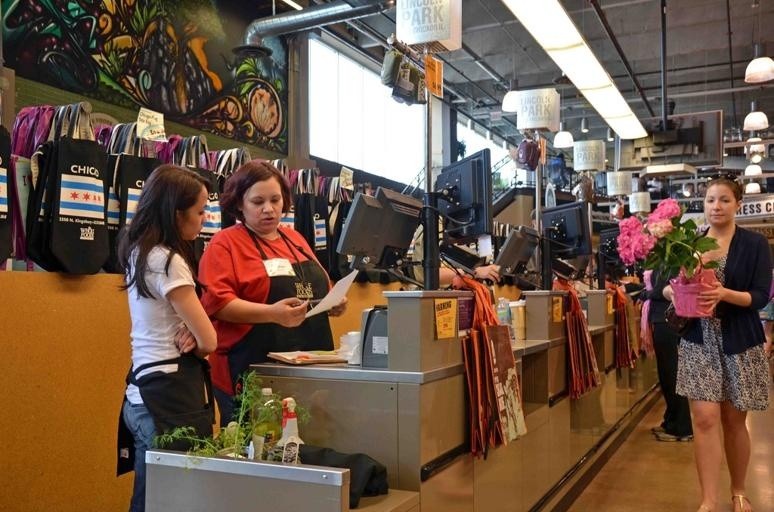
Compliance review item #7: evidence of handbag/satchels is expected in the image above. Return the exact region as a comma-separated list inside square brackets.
[267, 159, 353, 281]
[664, 302, 692, 337]
[515, 138, 541, 171]
[559, 290, 601, 402]
[95, 121, 164, 273]
[156, 133, 251, 263]
[2, 104, 110, 278]
[612, 288, 638, 369]
[452, 276, 530, 460]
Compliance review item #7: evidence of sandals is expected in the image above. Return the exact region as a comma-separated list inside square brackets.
[695, 503, 712, 512]
[731, 495, 752, 512]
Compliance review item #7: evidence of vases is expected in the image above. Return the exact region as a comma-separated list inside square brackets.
[668, 269, 718, 317]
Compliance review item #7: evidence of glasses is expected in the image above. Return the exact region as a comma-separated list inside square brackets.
[711, 174, 737, 179]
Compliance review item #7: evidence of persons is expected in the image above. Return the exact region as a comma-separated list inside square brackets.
[654, 175, 772, 512]
[196, 157, 350, 434]
[756, 244, 774, 363]
[409, 205, 505, 292]
[520, 206, 550, 279]
[113, 164, 218, 512]
[617, 249, 696, 444]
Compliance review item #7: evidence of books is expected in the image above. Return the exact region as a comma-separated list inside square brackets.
[266, 350, 349, 365]
[492, 220, 516, 237]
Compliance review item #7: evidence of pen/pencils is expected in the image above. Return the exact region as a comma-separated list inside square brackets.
[292, 299, 321, 306]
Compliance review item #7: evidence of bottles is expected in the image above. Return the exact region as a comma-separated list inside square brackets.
[251, 387, 281, 461]
[497, 296, 514, 338]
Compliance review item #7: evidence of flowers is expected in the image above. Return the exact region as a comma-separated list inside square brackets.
[616, 197, 717, 282]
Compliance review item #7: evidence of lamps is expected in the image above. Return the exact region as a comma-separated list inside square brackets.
[501, 23, 524, 112]
[581, 113, 589, 134]
[606, 128, 613, 143]
[501, 0, 648, 141]
[742, 0, 774, 193]
[553, 89, 574, 149]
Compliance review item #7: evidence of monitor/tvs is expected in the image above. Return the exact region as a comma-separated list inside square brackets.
[616, 109, 724, 171]
[336, 185, 425, 269]
[541, 200, 592, 259]
[600, 227, 634, 274]
[437, 148, 493, 245]
[494, 226, 537, 278]
[567, 255, 591, 280]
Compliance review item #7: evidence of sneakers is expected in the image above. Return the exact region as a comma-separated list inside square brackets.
[652, 426, 694, 442]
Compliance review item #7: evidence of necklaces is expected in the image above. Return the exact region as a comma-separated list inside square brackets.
[259, 232, 314, 301]
